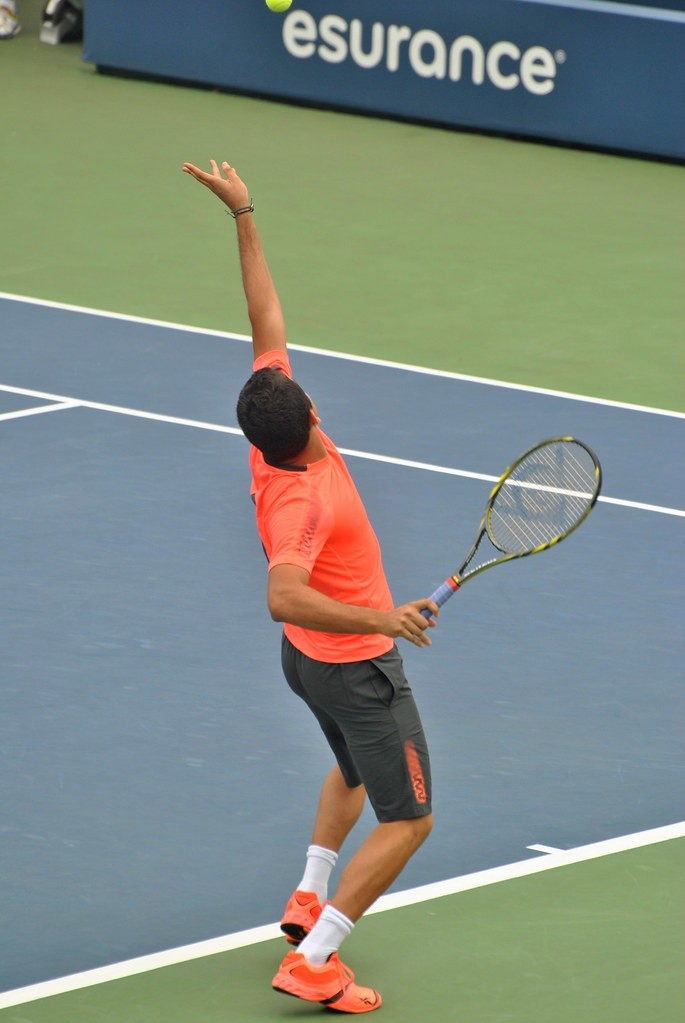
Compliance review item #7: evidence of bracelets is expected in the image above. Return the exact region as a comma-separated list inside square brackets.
[224, 198, 254, 218]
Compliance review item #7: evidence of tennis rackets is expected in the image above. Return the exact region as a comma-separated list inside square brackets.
[419, 437, 604, 620]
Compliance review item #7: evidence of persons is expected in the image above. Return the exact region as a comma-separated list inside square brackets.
[184, 158, 440, 1014]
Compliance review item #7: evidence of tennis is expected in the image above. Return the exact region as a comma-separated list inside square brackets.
[265, 0, 294, 14]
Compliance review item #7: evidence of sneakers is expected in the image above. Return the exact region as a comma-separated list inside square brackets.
[271, 950, 381, 1013]
[281, 890, 331, 947]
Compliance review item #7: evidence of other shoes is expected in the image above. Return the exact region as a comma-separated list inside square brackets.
[0, 8, 20, 38]
